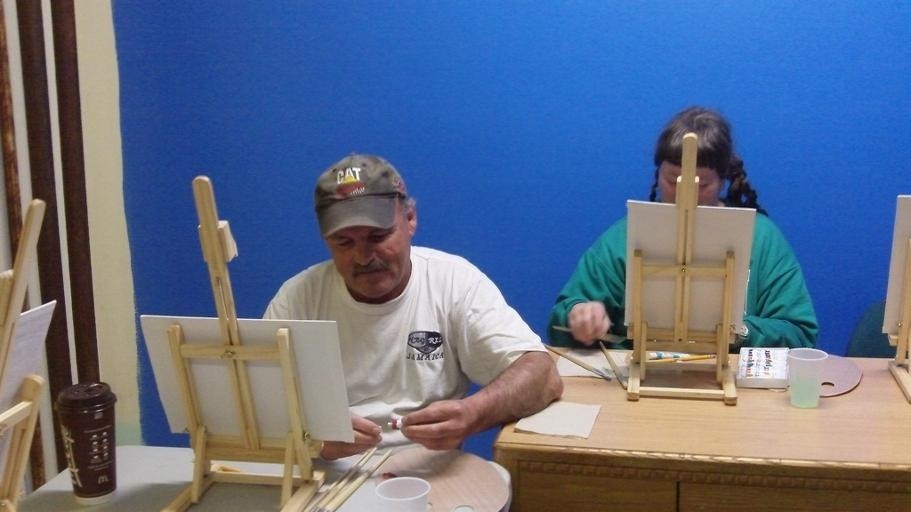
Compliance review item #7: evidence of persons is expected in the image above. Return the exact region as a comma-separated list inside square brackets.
[547, 105, 820, 349]
[257, 154, 563, 462]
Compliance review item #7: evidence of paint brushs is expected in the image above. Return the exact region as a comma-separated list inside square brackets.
[551, 325, 625, 344]
[599, 341, 628, 389]
[646, 354, 716, 364]
[543, 343, 611, 381]
[303, 446, 393, 512]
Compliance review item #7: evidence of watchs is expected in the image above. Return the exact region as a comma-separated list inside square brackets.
[732, 321, 749, 350]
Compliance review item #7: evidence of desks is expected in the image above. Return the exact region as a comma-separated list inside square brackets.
[13, 441, 511, 511]
[491, 339, 908, 512]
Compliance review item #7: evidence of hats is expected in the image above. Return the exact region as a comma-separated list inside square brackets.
[315, 150, 409, 238]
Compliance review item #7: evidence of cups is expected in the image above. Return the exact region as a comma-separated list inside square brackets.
[378, 478, 430, 511]
[787, 347, 829, 409]
[53, 383, 118, 504]
